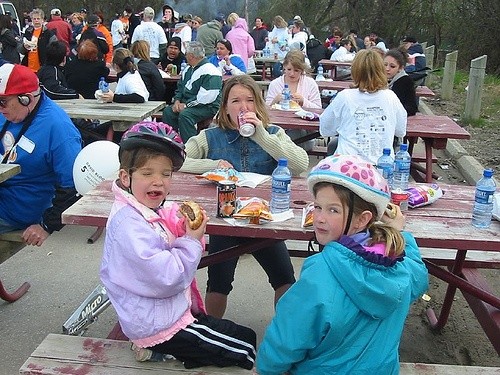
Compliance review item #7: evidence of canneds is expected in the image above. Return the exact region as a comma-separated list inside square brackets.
[172, 65, 177, 75]
[390, 188, 408, 216]
[216, 180, 237, 218]
[274, 52, 278, 59]
[237, 110, 255, 137]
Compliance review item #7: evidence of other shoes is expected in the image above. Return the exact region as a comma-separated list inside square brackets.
[128, 340, 152, 362]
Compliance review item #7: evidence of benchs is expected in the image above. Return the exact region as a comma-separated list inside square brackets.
[204, 235, 500, 269]
[18, 332, 500, 375]
[307, 146, 438, 163]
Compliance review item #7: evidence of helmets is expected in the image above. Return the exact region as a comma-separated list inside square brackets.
[307, 155, 391, 222]
[120, 121, 186, 161]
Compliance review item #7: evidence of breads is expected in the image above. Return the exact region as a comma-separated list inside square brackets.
[289, 100, 297, 109]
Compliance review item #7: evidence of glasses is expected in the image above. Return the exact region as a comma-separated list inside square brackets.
[0, 95, 17, 106]
[217, 39, 227, 43]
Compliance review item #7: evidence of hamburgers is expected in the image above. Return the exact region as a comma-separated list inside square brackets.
[385, 203, 397, 218]
[179, 201, 203, 229]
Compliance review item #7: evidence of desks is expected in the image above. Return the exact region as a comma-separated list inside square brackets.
[0, 57, 500, 330]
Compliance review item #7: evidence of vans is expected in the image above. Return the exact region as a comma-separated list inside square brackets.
[0, 2, 21, 41]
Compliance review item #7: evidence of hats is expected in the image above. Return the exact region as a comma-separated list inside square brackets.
[403, 38, 416, 43]
[142, 7, 155, 16]
[50, 9, 61, 15]
[167, 37, 181, 51]
[0, 63, 39, 96]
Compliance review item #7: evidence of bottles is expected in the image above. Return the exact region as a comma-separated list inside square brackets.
[99, 77, 108, 92]
[471, 169, 496, 228]
[269, 159, 292, 214]
[377, 148, 394, 190]
[267, 47, 270, 58]
[281, 85, 291, 109]
[317, 63, 323, 80]
[181, 60, 187, 72]
[263, 47, 267, 57]
[393, 144, 411, 190]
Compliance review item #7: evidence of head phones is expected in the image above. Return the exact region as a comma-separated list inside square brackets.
[17, 95, 30, 105]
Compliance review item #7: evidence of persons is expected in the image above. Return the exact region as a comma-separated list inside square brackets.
[181, 75, 309, 318]
[251, 153, 430, 375]
[251, 15, 316, 67]
[324, 27, 389, 79]
[0, 4, 255, 144]
[265, 50, 322, 151]
[384, 49, 417, 117]
[319, 49, 408, 168]
[1, 64, 82, 247]
[402, 35, 426, 86]
[101, 122, 257, 370]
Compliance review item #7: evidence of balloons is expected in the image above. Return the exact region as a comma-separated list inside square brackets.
[73, 141, 120, 196]
